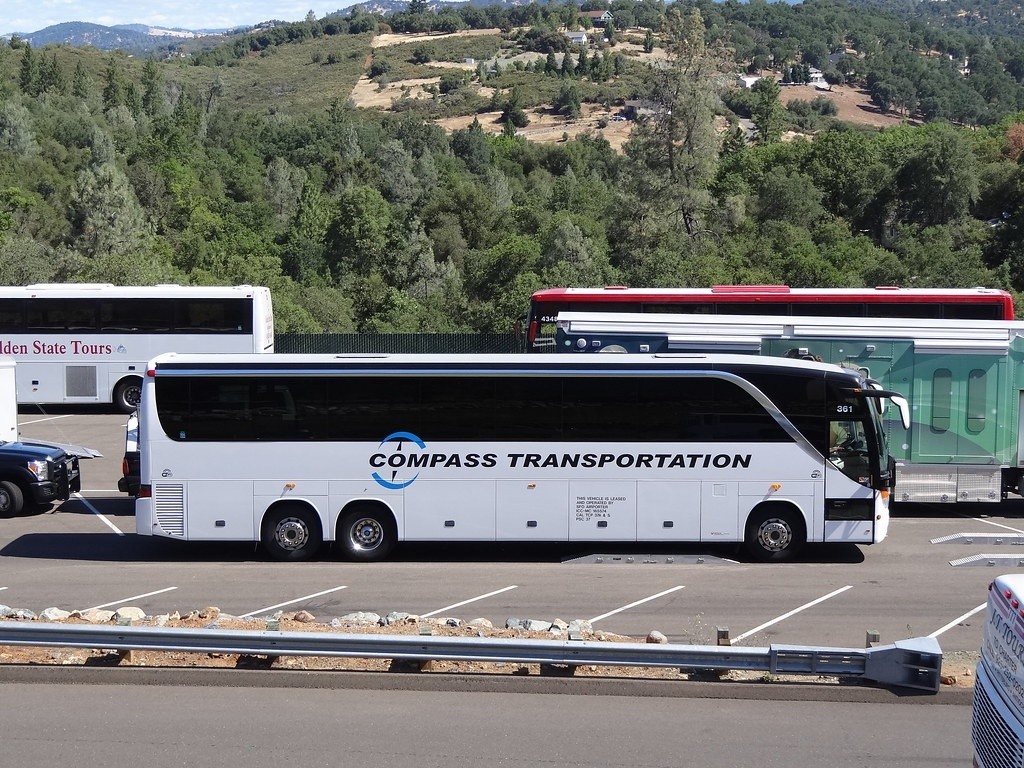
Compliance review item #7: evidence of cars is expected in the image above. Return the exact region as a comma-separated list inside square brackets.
[0, 437, 81, 518]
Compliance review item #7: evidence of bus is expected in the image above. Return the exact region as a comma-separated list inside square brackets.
[0, 283, 275, 415]
[118, 352, 910, 563]
[513, 284, 1013, 353]
